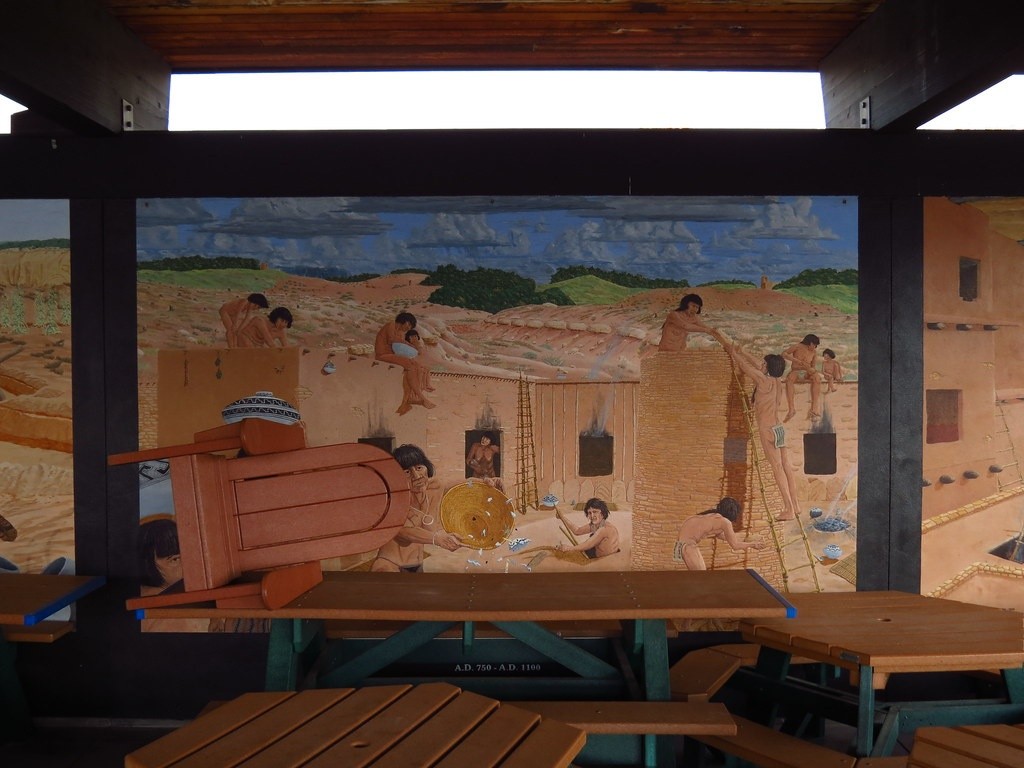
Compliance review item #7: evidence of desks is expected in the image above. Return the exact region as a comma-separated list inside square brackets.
[125, 685, 585, 768]
[136, 570, 797, 768]
[0, 621, 75, 643]
[740, 590, 1024, 767]
[0, 571, 107, 744]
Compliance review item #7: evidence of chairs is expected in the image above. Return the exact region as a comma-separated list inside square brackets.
[106, 416, 413, 612]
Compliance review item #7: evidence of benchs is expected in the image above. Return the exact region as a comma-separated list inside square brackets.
[193, 701, 739, 735]
[670, 642, 1004, 767]
[323, 619, 624, 640]
